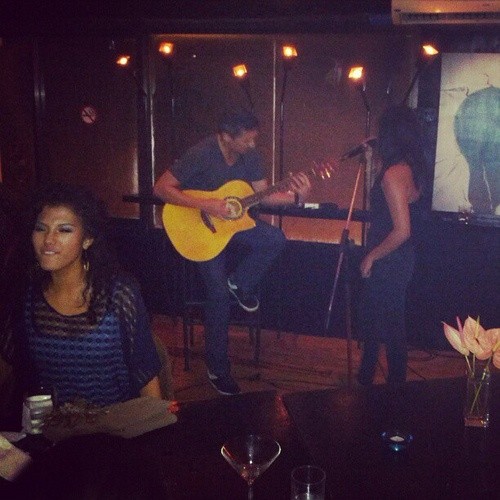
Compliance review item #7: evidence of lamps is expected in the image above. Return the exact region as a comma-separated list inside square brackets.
[278, 45, 298, 229]
[159, 41, 178, 160]
[232, 64, 255, 112]
[347, 66, 371, 247]
[403, 44, 437, 106]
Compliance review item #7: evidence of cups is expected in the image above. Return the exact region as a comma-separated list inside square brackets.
[458, 205, 471, 222]
[26, 395, 53, 434]
[290, 465, 326, 500]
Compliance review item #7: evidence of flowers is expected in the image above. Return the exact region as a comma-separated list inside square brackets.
[443, 312, 500, 416]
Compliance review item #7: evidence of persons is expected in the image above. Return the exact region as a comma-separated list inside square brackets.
[350, 103, 426, 384]
[154, 111, 311, 396]
[3, 188, 162, 406]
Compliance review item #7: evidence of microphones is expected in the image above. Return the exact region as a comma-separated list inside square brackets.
[339, 137, 376, 161]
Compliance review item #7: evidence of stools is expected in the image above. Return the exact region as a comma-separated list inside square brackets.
[180, 258, 263, 370]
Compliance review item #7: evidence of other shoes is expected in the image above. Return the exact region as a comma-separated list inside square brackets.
[341, 376, 370, 388]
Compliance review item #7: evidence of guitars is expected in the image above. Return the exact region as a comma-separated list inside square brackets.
[161, 157, 339, 262]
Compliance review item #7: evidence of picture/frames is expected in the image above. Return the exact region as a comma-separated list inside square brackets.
[421, 45, 500, 228]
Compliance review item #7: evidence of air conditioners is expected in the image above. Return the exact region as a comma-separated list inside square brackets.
[391, 0, 500, 26]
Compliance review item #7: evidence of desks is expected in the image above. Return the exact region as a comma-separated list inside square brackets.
[282, 372, 500, 500]
[0, 388, 328, 500]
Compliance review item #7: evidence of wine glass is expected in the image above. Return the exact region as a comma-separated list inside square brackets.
[221, 434, 281, 500]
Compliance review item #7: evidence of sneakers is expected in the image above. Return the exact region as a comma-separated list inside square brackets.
[226, 276, 260, 312]
[207, 370, 243, 396]
